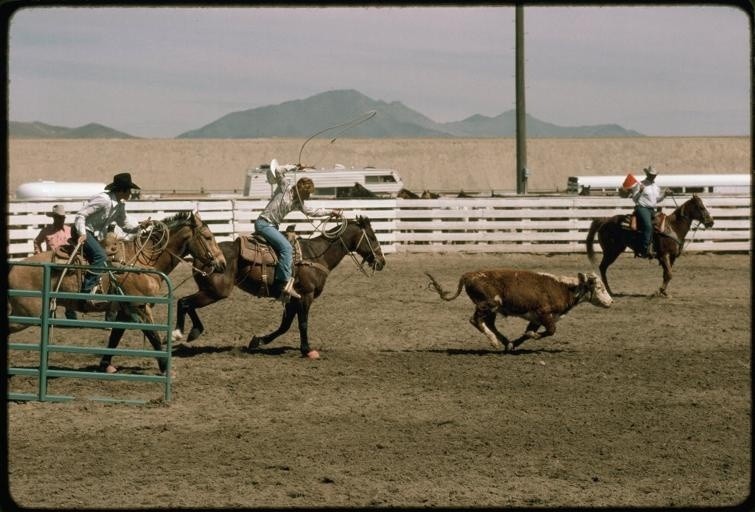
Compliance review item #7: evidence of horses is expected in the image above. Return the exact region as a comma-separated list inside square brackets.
[162, 213, 386, 360]
[342, 182, 591, 245]
[7, 203, 227, 378]
[586, 192, 715, 297]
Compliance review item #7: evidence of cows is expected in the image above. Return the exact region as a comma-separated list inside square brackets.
[423, 267, 614, 350]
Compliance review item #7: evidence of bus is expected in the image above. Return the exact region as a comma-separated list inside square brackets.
[565, 172, 753, 196]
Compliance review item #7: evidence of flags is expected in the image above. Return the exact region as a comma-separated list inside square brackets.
[623, 174, 641, 197]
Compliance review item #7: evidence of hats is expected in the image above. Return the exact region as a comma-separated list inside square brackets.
[104, 173, 141, 190]
[46, 205, 67, 217]
[644, 165, 659, 175]
[266, 159, 279, 184]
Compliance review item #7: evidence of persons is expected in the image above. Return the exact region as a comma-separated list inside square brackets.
[34, 205, 77, 319]
[255, 163, 342, 298]
[71, 173, 152, 312]
[632, 165, 672, 257]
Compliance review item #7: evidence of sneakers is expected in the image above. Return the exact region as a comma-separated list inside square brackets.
[280, 281, 301, 298]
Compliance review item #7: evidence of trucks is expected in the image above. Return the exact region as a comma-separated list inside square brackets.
[246, 163, 404, 200]
